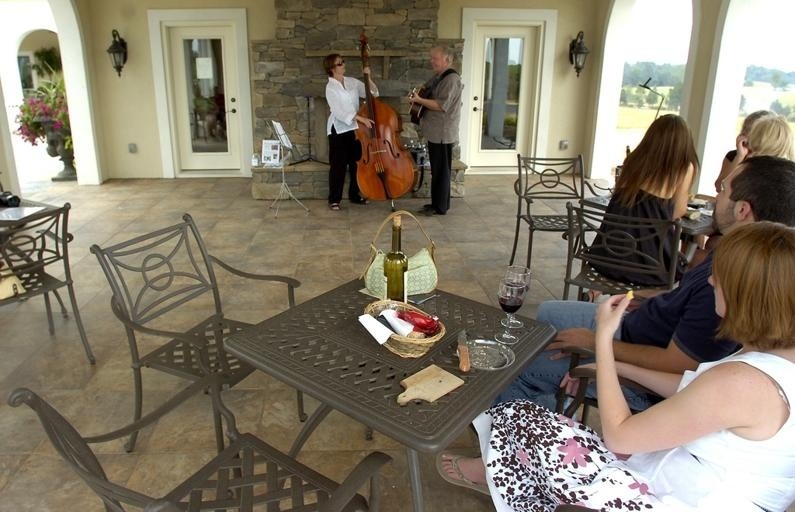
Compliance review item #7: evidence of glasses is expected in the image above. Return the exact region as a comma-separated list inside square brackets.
[332, 59, 345, 68]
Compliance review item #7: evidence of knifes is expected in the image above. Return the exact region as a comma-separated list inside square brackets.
[457, 329, 472, 372]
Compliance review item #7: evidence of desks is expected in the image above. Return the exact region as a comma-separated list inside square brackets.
[0, 193, 65, 334]
[223, 276, 558, 511]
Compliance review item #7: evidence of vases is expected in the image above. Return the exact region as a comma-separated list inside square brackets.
[50, 127, 77, 184]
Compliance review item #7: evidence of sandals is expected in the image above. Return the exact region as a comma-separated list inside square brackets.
[330, 201, 341, 211]
[353, 197, 371, 205]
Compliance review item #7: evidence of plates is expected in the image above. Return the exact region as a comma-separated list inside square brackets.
[461, 338, 516, 372]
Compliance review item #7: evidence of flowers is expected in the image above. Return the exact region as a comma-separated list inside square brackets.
[12, 60, 75, 151]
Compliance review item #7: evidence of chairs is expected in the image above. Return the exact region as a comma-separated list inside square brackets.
[550, 367, 668, 512]
[561, 344, 643, 429]
[90, 213, 308, 451]
[562, 152, 718, 305]
[508, 154, 602, 271]
[0, 203, 96, 363]
[6, 388, 392, 509]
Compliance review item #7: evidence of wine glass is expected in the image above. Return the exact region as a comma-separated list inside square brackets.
[493, 261, 532, 345]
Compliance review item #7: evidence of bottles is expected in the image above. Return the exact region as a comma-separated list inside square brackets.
[624, 145, 630, 157]
[382, 212, 408, 300]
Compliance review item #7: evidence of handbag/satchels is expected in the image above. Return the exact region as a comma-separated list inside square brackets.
[363, 209, 439, 299]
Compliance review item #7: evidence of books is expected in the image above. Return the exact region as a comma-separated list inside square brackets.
[273, 119, 294, 150]
[261, 138, 281, 164]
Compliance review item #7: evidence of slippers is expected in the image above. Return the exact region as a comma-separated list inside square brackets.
[438, 450, 491, 497]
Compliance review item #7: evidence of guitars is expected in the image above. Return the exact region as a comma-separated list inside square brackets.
[408, 87, 429, 125]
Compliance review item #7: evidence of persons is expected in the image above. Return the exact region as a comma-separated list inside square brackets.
[442, 219, 794, 512]
[405, 43, 464, 216]
[747, 113, 793, 163]
[585, 109, 699, 290]
[485, 154, 793, 420]
[712, 107, 795, 197]
[321, 53, 381, 211]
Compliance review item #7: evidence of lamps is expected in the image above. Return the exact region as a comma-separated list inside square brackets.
[105, 27, 133, 79]
[568, 29, 590, 79]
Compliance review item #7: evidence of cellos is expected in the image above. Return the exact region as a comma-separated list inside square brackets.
[354, 34, 418, 212]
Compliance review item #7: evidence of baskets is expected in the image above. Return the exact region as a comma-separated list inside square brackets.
[365, 299, 446, 360]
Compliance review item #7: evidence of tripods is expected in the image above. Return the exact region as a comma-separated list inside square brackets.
[269, 147, 311, 219]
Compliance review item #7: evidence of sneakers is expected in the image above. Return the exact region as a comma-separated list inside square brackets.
[418, 203, 450, 216]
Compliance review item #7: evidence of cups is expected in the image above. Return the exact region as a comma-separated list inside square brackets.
[614, 164, 624, 187]
